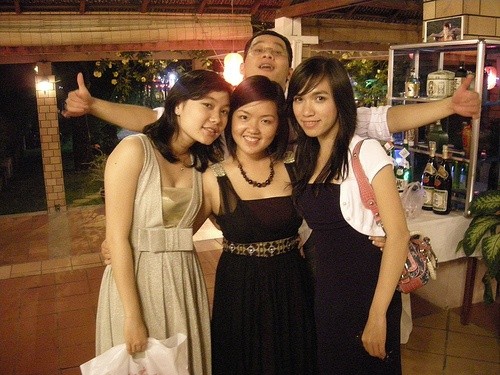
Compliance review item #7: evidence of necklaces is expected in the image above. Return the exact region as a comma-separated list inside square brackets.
[231, 150, 276, 188]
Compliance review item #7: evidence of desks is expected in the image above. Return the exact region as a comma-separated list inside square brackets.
[398, 191, 490, 344]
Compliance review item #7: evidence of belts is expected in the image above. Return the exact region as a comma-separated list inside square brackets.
[223, 236, 296, 257]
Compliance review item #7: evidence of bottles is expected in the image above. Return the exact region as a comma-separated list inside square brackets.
[388, 120, 468, 215]
[454, 61, 466, 94]
[427, 70, 456, 99]
[405, 66, 421, 99]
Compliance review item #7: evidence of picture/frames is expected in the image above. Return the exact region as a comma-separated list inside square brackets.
[424, 14, 463, 43]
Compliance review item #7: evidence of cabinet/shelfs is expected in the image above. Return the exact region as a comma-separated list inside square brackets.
[387, 38, 500, 217]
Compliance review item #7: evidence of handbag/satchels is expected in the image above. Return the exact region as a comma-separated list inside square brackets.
[80, 333, 187, 375]
[352, 137, 438, 294]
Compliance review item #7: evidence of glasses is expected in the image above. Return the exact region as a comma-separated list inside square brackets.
[247, 47, 289, 57]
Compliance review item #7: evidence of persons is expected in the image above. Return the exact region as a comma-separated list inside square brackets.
[63, 29, 480, 143]
[95, 70, 306, 375]
[101, 76, 388, 375]
[284, 56, 409, 375]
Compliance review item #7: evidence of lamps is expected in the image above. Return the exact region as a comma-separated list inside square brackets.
[39, 72, 51, 96]
[221, 1, 245, 86]
[483, 66, 497, 89]
